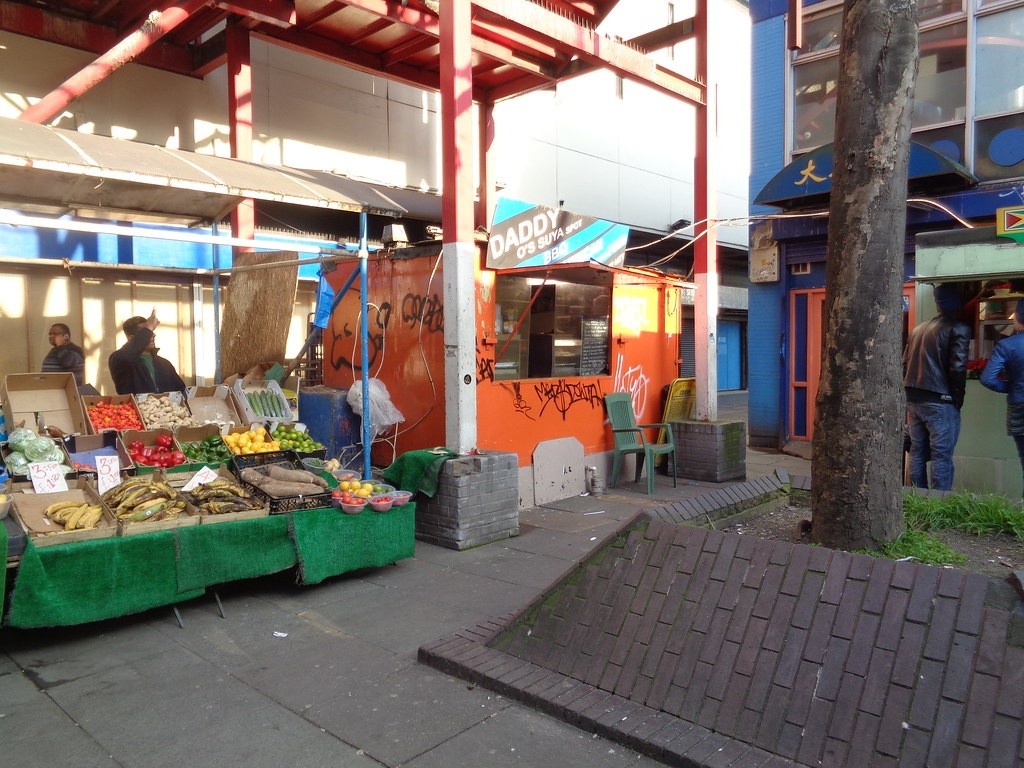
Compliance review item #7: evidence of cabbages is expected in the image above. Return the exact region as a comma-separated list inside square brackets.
[4, 429, 71, 473]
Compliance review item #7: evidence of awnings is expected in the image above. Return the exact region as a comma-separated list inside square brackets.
[752, 135, 980, 208]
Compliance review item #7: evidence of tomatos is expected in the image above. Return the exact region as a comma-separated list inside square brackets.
[333, 491, 363, 505]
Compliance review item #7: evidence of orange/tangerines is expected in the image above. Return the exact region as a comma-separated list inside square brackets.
[374, 485, 393, 494]
[272, 426, 322, 452]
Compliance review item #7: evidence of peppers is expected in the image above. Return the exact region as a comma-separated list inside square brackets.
[128, 434, 184, 468]
[179, 435, 231, 463]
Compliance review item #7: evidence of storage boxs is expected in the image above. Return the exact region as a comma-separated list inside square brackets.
[80, 394, 147, 435]
[161, 462, 271, 525]
[243, 481, 333, 516]
[233, 378, 293, 426]
[266, 420, 328, 461]
[173, 422, 233, 471]
[0, 372, 87, 435]
[228, 448, 308, 488]
[87, 469, 201, 537]
[0, 438, 77, 483]
[134, 391, 191, 418]
[120, 427, 190, 476]
[60, 430, 136, 481]
[5, 474, 118, 548]
[183, 384, 242, 425]
[220, 421, 288, 468]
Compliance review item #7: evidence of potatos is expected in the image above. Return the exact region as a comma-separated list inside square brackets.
[241, 465, 330, 507]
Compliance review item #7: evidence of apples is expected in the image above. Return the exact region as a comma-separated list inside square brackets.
[339, 481, 373, 497]
[225, 427, 280, 454]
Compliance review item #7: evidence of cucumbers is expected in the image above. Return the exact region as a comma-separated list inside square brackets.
[246, 390, 286, 418]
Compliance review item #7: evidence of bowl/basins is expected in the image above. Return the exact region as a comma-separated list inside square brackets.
[339, 498, 368, 515]
[368, 494, 394, 512]
[388, 490, 413, 507]
[302, 458, 325, 475]
[371, 484, 396, 498]
[331, 470, 362, 483]
[0, 483, 7, 493]
[331, 496, 342, 507]
[0, 493, 13, 519]
[361, 479, 382, 486]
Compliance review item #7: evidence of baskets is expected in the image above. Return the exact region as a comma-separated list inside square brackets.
[230, 448, 333, 514]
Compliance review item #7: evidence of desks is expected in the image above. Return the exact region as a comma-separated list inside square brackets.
[7, 501, 416, 628]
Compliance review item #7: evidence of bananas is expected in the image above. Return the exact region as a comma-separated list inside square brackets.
[45, 477, 263, 532]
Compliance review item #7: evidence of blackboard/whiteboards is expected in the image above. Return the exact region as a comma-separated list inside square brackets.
[579, 315, 610, 376]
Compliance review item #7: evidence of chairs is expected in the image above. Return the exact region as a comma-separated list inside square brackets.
[603, 393, 678, 495]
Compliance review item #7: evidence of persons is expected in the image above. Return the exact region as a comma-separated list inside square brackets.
[979, 297, 1024, 501]
[900, 281, 971, 491]
[109, 307, 187, 394]
[42, 324, 85, 387]
